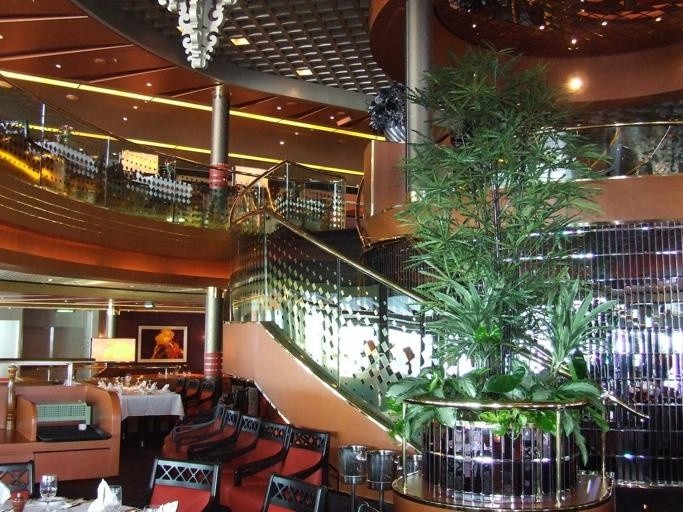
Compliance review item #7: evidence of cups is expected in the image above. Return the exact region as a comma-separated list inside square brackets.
[104, 486, 122, 511]
[143, 505, 163, 511]
[79, 424, 87, 431]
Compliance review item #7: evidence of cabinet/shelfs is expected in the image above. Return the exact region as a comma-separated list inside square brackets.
[31, 447, 113, 484]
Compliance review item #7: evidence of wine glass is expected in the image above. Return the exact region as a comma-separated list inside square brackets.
[41, 475, 57, 511]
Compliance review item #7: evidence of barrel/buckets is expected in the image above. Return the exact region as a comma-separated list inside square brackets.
[335, 444, 368, 485]
[393, 454, 423, 478]
[365, 449, 396, 490]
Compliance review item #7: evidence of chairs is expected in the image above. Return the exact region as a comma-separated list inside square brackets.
[129, 377, 330, 511]
[0, 458, 35, 501]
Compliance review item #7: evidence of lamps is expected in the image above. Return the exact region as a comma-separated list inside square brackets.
[89, 336, 136, 377]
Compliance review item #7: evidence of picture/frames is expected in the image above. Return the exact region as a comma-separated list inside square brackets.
[136, 325, 188, 364]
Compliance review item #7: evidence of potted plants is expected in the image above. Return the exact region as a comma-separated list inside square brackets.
[387, 38, 622, 510]
